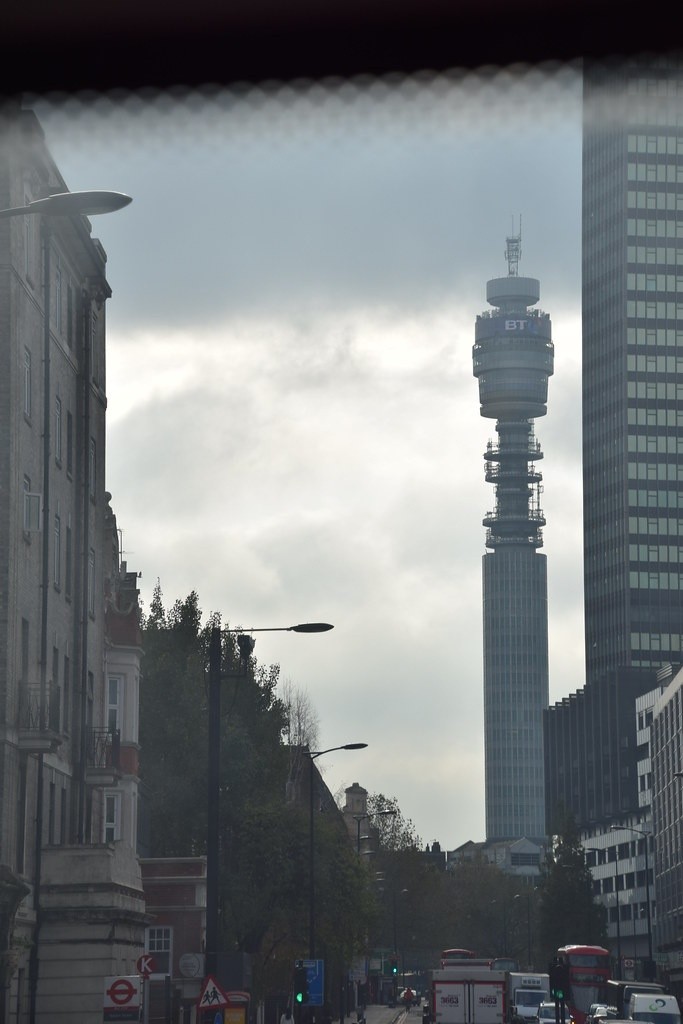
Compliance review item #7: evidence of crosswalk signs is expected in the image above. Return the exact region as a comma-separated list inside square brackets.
[195, 974, 231, 1012]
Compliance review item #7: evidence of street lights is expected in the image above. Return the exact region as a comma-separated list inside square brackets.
[298, 744, 370, 1024]
[351, 810, 398, 1012]
[204, 624, 336, 1023]
[610, 825, 662, 983]
[514, 893, 533, 972]
[587, 847, 629, 980]
[491, 899, 507, 958]
[388, 888, 409, 1007]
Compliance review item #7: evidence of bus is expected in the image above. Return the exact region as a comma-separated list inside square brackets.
[441, 949, 476, 959]
[549, 945, 612, 1024]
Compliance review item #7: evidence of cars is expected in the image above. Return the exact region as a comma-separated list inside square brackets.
[535, 1002, 572, 1024]
[396, 989, 422, 1007]
[586, 1003, 609, 1024]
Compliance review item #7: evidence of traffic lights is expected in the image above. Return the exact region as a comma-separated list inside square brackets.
[294, 967, 311, 1005]
[550, 957, 569, 1002]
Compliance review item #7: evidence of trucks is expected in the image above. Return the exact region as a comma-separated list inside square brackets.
[422, 960, 507, 1024]
[507, 971, 552, 1024]
[604, 979, 683, 1024]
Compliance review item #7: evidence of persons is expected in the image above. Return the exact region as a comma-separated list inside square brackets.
[404, 987, 414, 1013]
[280, 1007, 295, 1024]
